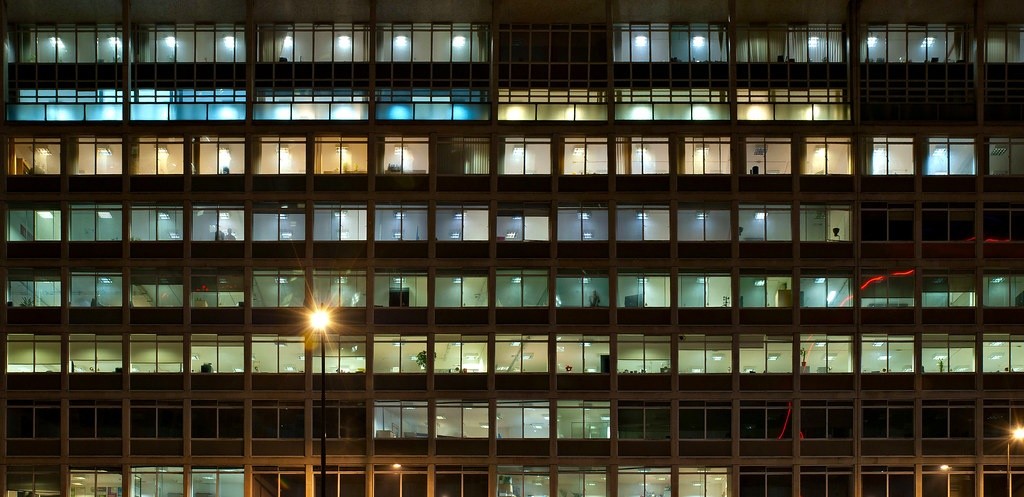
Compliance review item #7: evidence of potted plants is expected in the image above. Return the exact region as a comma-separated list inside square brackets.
[415, 350, 437, 370]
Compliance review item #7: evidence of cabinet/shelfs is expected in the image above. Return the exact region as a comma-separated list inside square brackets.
[376, 430, 393, 438]
[498, 484, 513, 493]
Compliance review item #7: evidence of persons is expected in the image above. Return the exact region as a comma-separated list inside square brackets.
[224, 228, 237, 241]
